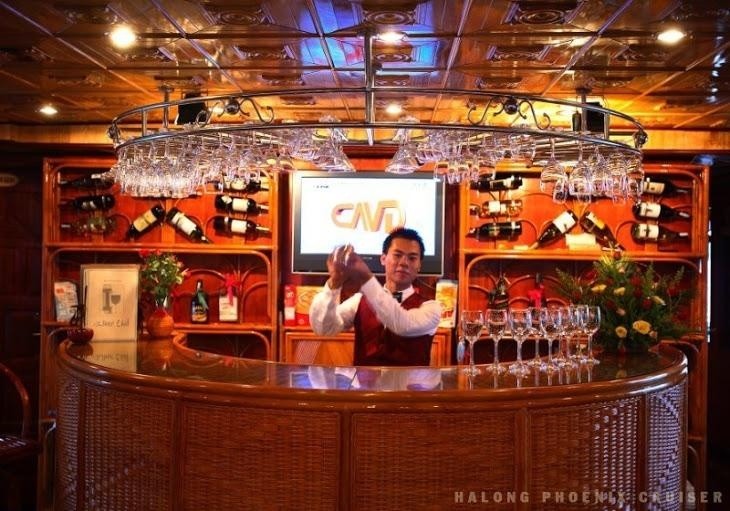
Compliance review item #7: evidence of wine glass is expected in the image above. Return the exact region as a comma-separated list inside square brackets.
[526, 304, 601, 373]
[484, 309, 509, 376]
[108, 116, 356, 198]
[508, 308, 532, 375]
[383, 129, 645, 202]
[461, 310, 483, 377]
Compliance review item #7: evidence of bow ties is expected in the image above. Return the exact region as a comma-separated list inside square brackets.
[392, 290, 402, 303]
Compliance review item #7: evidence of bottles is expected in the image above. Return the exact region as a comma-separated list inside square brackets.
[214, 172, 271, 192]
[493, 279, 509, 330]
[214, 215, 271, 238]
[191, 281, 209, 325]
[120, 206, 165, 243]
[466, 176, 691, 250]
[132, 189, 204, 201]
[60, 218, 118, 237]
[58, 173, 114, 191]
[215, 195, 271, 215]
[58, 192, 114, 215]
[164, 208, 213, 245]
[528, 272, 551, 332]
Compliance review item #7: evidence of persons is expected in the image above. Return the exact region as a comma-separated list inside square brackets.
[309, 228, 440, 366]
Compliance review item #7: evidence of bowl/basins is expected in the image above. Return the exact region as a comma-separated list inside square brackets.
[66, 327, 94, 344]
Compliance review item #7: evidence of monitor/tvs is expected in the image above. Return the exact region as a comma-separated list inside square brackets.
[291, 168, 447, 278]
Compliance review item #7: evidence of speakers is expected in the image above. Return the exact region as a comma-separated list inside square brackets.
[587, 110, 604, 133]
[176, 101, 206, 125]
